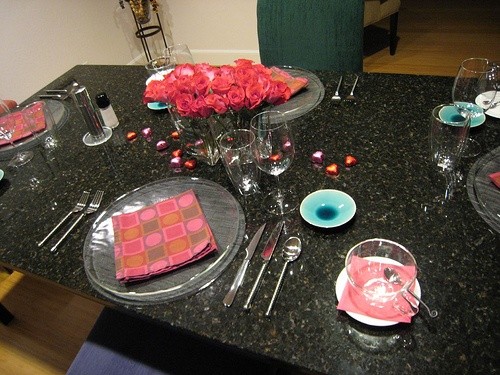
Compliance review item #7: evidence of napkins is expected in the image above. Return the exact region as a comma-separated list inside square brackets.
[0, 102, 48, 146]
[334, 255, 419, 323]
[269, 66, 309, 101]
[110, 189, 219, 282]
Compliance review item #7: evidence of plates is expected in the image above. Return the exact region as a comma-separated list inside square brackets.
[299, 189, 356, 227]
[0, 100, 70, 160]
[82, 175, 246, 305]
[467, 148, 500, 234]
[475, 90, 500, 118]
[254, 64, 325, 125]
[335, 256, 422, 327]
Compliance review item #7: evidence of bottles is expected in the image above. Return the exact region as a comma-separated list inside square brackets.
[71, 83, 105, 140]
[96, 93, 119, 129]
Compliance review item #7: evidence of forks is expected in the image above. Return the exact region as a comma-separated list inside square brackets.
[330, 74, 360, 108]
[37, 191, 104, 254]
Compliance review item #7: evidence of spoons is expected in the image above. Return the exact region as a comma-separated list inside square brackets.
[384, 267, 439, 319]
[264, 236, 302, 316]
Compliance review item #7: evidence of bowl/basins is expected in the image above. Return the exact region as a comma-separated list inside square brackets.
[345, 238, 418, 304]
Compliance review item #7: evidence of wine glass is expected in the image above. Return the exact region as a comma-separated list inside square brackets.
[444, 57, 498, 159]
[0, 101, 34, 167]
[249, 110, 300, 216]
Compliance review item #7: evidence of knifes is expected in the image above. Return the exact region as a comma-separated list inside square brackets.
[222, 223, 267, 308]
[243, 221, 283, 312]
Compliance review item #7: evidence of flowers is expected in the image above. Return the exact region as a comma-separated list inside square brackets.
[142, 58, 291, 117]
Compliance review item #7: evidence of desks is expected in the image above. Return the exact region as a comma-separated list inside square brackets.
[0, 65, 500, 375]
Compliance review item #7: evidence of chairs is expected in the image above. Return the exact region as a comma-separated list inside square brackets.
[363, 0, 405, 56]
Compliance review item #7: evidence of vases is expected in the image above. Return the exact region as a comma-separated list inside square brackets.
[163, 102, 278, 168]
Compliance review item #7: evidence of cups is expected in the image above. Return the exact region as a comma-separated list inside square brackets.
[219, 128, 260, 197]
[429, 103, 472, 175]
[21, 100, 61, 150]
[144, 56, 177, 75]
[165, 43, 194, 64]
